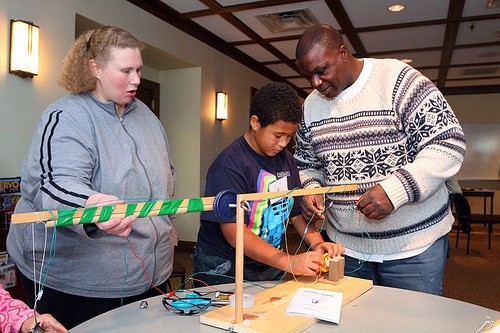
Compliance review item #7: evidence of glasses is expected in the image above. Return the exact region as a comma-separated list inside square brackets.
[162, 297, 211, 316]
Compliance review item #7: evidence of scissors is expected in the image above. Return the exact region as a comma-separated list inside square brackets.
[172, 293, 228, 308]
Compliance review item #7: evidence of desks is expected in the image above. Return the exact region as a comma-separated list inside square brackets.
[454, 187, 495, 250]
[68, 277, 500, 333]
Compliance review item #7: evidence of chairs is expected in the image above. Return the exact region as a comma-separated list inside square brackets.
[451, 193, 471, 256]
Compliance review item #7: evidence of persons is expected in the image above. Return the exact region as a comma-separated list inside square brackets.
[6, 25, 179, 330]
[193, 82, 346, 287]
[0, 283, 68, 333]
[294, 24, 467, 295]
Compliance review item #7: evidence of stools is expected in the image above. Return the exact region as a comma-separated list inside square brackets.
[167, 258, 186, 289]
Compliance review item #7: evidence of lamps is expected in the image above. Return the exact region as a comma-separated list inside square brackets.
[8, 18, 40, 80]
[217, 91, 229, 122]
